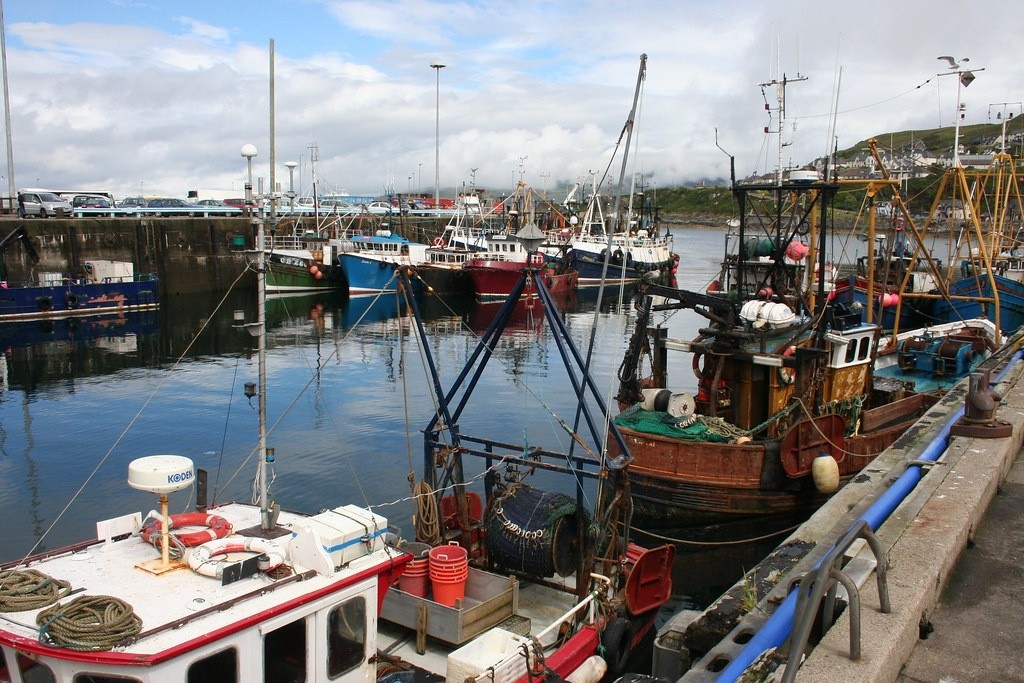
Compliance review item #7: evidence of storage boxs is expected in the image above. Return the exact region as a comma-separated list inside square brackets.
[379, 566, 518, 645]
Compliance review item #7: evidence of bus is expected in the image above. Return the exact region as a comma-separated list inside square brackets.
[15, 187, 116, 217]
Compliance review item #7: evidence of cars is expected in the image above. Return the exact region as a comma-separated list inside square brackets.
[368, 201, 399, 216]
[194, 199, 241, 216]
[223, 199, 260, 215]
[147, 197, 206, 217]
[118, 197, 149, 217]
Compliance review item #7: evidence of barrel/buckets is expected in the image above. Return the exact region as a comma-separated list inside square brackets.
[398, 540, 469, 606]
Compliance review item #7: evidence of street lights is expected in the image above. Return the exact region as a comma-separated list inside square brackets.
[408, 163, 424, 198]
[431, 63, 446, 209]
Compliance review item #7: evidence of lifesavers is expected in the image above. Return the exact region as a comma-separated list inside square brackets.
[599, 248, 674, 275]
[691, 352, 705, 379]
[433, 237, 445, 247]
[547, 278, 553, 288]
[890, 216, 906, 232]
[568, 275, 573, 285]
[65, 293, 78, 306]
[379, 261, 387, 269]
[778, 345, 797, 384]
[140, 512, 233, 548]
[37, 296, 52, 310]
[188, 536, 287, 579]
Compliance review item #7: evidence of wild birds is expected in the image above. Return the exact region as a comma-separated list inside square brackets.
[937, 55, 970, 72]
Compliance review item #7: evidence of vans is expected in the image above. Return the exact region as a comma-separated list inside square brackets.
[298, 196, 315, 206]
[317, 199, 353, 217]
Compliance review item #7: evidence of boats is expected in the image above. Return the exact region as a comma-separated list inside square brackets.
[1, 259, 161, 323]
[0, 172, 670, 683]
[601, 34, 1024, 548]
[246, 36, 679, 305]
